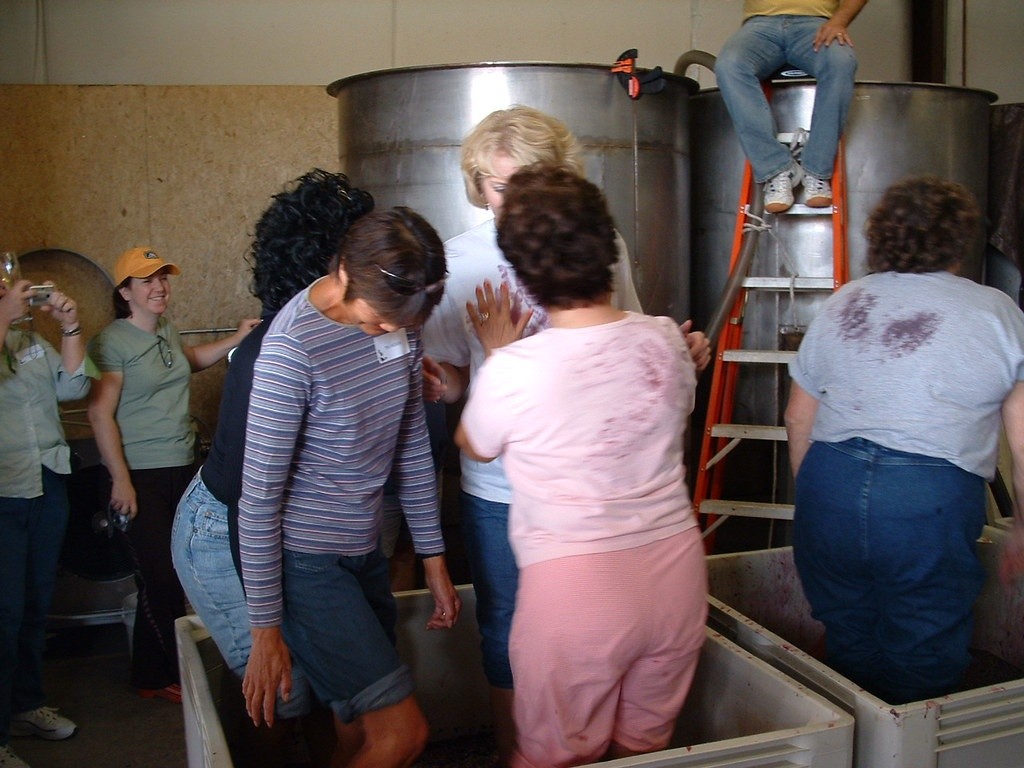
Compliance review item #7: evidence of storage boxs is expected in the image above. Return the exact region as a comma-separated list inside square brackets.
[175, 542, 1024, 768]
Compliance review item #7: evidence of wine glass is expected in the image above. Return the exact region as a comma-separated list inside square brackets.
[0, 252, 33, 324]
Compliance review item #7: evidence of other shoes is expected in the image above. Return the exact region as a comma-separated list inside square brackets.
[140, 678, 184, 703]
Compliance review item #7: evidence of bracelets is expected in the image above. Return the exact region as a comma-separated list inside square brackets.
[61, 325, 82, 337]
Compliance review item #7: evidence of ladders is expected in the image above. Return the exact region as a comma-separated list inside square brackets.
[691, 82, 849, 556]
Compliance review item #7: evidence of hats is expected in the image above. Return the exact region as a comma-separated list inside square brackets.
[115, 247, 180, 288]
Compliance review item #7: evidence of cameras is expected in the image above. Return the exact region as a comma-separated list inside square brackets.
[112, 512, 132, 533]
[29, 285, 55, 306]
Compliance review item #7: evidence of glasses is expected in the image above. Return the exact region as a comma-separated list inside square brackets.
[157, 335, 173, 368]
[375, 263, 450, 295]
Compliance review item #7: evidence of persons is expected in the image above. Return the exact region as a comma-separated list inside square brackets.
[171, 167, 376, 768]
[422, 107, 710, 768]
[0, 271, 102, 768]
[715, 0, 868, 213]
[785, 175, 1024, 706]
[238, 206, 462, 768]
[455, 163, 707, 768]
[87, 247, 261, 703]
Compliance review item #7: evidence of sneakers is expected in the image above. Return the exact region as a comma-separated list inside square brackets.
[8, 703, 78, 741]
[763, 159, 804, 213]
[0, 743, 31, 768]
[802, 170, 834, 208]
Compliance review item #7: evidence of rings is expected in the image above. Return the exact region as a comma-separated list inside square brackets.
[442, 611, 446, 616]
[837, 33, 843, 36]
[481, 312, 490, 323]
